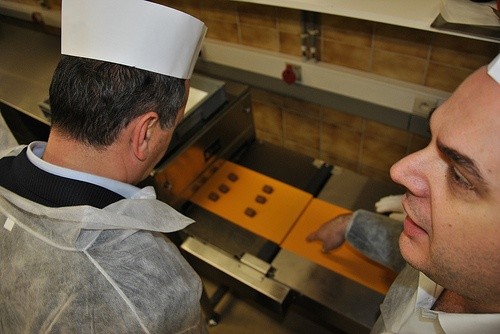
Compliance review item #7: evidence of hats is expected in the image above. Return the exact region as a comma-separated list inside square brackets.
[61, 0, 208, 80]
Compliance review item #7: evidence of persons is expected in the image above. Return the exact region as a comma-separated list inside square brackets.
[0, 0, 203, 334]
[305, 51, 500, 334]
